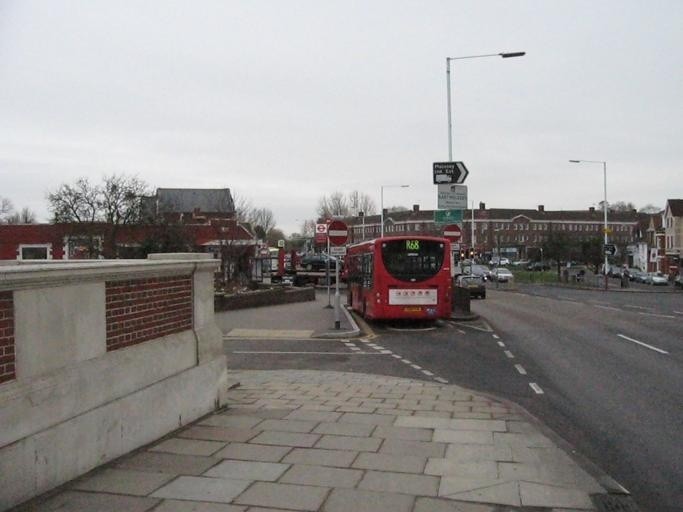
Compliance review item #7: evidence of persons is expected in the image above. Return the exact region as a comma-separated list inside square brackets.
[623, 266, 632, 288]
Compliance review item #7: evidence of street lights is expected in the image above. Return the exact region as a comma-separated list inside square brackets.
[447, 53, 524, 163]
[570, 160, 608, 288]
[380, 185, 409, 238]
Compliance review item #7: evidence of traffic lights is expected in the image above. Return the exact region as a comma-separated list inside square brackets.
[470, 248, 474, 258]
[461, 250, 464, 260]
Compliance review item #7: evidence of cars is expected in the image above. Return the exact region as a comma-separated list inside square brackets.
[454, 257, 550, 299]
[300, 255, 344, 272]
[601, 264, 668, 286]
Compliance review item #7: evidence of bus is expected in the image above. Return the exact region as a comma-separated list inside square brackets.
[261, 247, 286, 271]
[343, 236, 453, 320]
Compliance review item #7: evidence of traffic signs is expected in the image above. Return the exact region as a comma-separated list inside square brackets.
[434, 209, 463, 224]
[601, 244, 618, 256]
[438, 184, 467, 209]
[433, 162, 468, 184]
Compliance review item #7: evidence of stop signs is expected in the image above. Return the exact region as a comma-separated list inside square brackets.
[328, 221, 348, 246]
[444, 224, 462, 242]
[316, 224, 328, 233]
